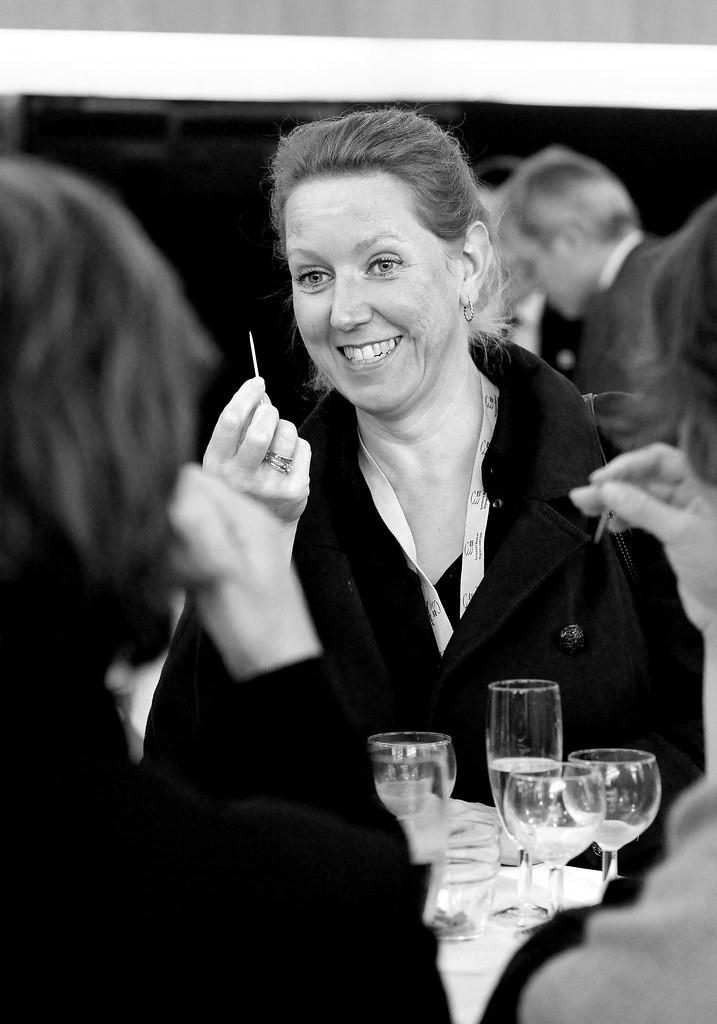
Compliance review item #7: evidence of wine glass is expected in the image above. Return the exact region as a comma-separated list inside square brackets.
[563, 748, 663, 905]
[503, 762, 607, 944]
[487, 678, 564, 927]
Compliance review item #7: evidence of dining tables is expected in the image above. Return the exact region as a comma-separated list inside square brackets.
[423, 862, 622, 1024]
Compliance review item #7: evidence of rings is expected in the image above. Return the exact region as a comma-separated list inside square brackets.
[265, 447, 291, 473]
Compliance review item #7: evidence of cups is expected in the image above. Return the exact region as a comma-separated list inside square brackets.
[435, 815, 502, 942]
[367, 731, 457, 817]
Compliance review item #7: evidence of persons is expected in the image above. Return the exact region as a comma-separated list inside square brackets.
[174, 107, 703, 880]
[492, 149, 717, 482]
[470, 199, 708, 1024]
[1, 148, 457, 1024]
